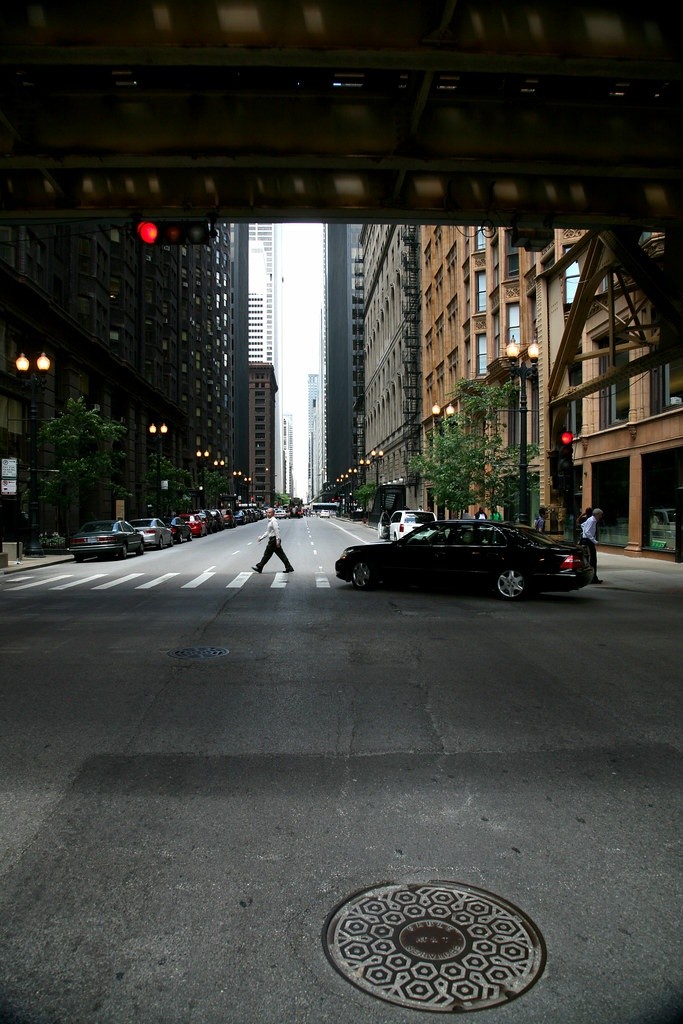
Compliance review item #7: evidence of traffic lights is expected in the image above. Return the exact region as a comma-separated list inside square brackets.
[557, 430, 574, 476]
[134, 217, 214, 248]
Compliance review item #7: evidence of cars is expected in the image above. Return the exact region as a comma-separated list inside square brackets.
[334, 517, 594, 602]
[68, 497, 310, 562]
[648, 508, 676, 540]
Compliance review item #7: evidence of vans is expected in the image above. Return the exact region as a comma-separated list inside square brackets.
[378, 509, 437, 542]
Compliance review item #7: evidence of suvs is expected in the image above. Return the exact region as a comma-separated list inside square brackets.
[320, 510, 330, 518]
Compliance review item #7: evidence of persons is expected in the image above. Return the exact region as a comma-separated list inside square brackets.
[403, 505, 431, 511]
[649, 511, 661, 538]
[250, 508, 295, 573]
[576, 508, 604, 583]
[535, 507, 548, 533]
[462, 506, 504, 521]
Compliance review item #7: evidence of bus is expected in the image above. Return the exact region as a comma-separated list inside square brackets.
[311, 503, 338, 514]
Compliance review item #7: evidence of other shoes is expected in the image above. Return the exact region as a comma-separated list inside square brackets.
[252, 566, 262, 573]
[592, 580, 602, 584]
[283, 568, 294, 573]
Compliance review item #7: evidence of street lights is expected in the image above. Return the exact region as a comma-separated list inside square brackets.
[370, 450, 384, 516]
[196, 449, 209, 510]
[431, 401, 456, 521]
[358, 459, 370, 522]
[16, 351, 52, 559]
[506, 334, 539, 531]
[213, 459, 252, 507]
[335, 468, 357, 518]
[146, 423, 169, 519]
